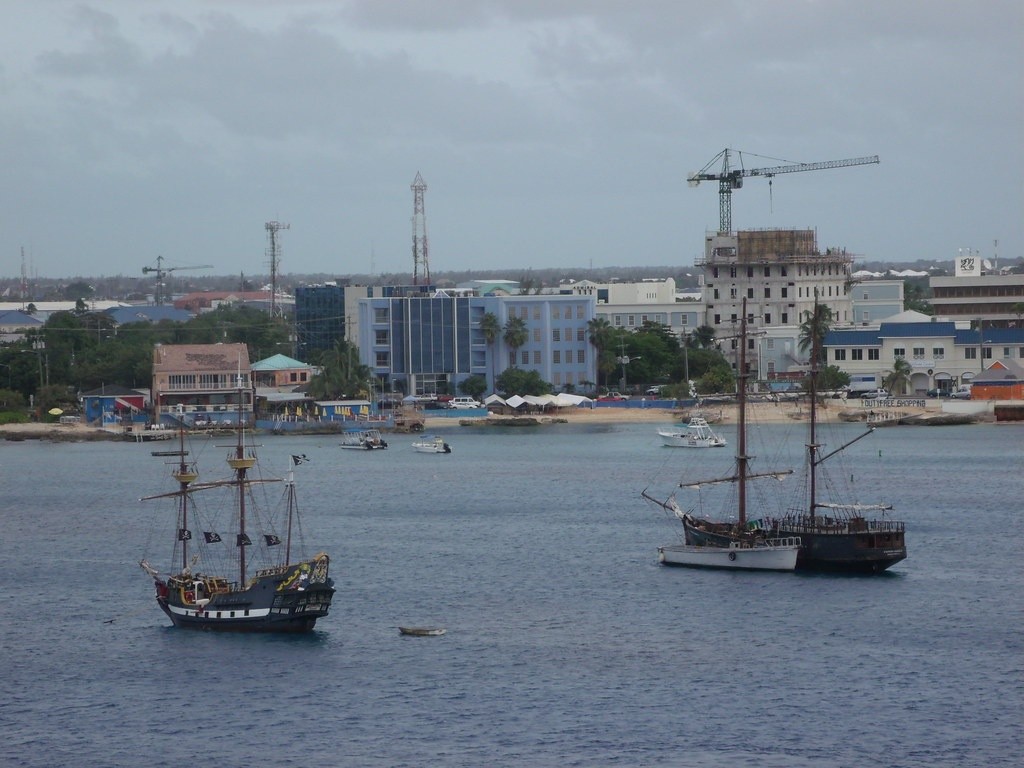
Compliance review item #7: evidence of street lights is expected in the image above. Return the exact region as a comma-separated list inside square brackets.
[21, 349, 43, 387]
[97, 319, 106, 345]
[391, 378, 397, 408]
[624, 356, 642, 394]
[277, 342, 307, 359]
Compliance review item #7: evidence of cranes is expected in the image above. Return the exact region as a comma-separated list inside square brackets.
[686, 147, 881, 259]
[142, 255, 216, 306]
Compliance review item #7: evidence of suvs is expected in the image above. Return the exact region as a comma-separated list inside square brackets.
[417, 393, 438, 403]
[449, 395, 481, 408]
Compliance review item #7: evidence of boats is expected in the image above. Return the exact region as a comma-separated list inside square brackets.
[657, 416, 729, 448]
[655, 535, 804, 572]
[397, 625, 446, 637]
[149, 449, 191, 457]
[865, 408, 925, 427]
[338, 428, 388, 451]
[251, 398, 344, 436]
[410, 435, 453, 454]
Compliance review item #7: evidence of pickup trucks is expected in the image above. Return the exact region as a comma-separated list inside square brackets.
[599, 391, 631, 402]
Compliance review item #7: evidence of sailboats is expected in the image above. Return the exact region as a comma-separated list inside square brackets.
[135, 349, 340, 635]
[640, 291, 909, 575]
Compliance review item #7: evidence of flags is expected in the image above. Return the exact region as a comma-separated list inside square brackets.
[236, 534, 252, 546]
[179, 529, 192, 541]
[292, 454, 309, 466]
[203, 532, 222, 543]
[264, 534, 282, 546]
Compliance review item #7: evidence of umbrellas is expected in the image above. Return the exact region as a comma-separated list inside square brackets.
[48, 408, 63, 426]
[363, 405, 368, 421]
[323, 408, 327, 422]
[314, 407, 318, 422]
[334, 406, 351, 421]
[284, 407, 289, 422]
[296, 407, 302, 422]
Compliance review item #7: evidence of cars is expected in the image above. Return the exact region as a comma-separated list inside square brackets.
[378, 398, 401, 408]
[950, 388, 971, 399]
[861, 388, 892, 399]
[596, 395, 622, 402]
[450, 401, 478, 409]
[424, 399, 451, 410]
[439, 395, 454, 402]
[926, 388, 950, 397]
[646, 386, 660, 395]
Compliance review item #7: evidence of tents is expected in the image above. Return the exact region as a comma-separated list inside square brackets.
[484, 393, 592, 417]
